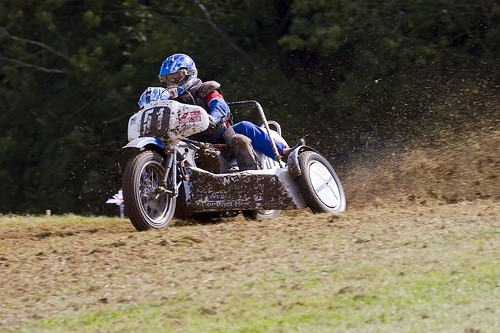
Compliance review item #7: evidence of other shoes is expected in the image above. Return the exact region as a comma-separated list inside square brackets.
[281, 139, 305, 163]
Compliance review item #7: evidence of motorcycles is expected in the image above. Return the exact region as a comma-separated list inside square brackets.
[123, 85, 346, 231]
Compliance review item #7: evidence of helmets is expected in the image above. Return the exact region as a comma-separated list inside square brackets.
[138, 87, 172, 109]
[159, 53, 198, 98]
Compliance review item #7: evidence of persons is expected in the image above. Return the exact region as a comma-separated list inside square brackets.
[159, 54, 306, 163]
